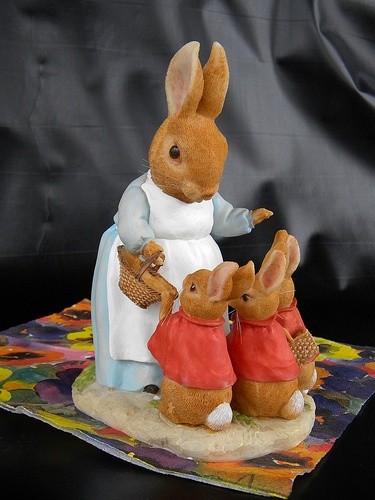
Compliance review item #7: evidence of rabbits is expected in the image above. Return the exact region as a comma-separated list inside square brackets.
[89, 39, 319, 432]
